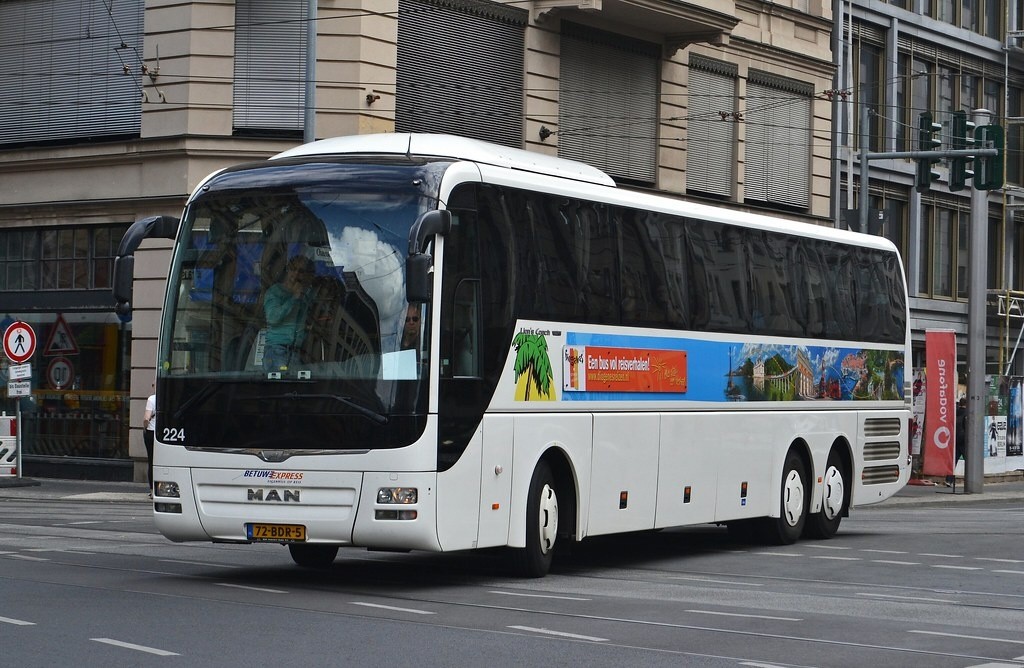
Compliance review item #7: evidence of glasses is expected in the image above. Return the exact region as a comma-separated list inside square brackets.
[401, 316, 419, 323]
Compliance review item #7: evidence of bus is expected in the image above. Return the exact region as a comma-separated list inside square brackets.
[113, 132, 915, 581]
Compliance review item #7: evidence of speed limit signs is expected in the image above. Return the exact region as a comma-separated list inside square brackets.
[48, 358, 75, 391]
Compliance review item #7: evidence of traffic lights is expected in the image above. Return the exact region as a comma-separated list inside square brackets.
[949, 111, 975, 193]
[917, 111, 942, 188]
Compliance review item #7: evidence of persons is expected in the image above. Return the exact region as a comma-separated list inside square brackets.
[143, 379, 156, 500]
[262, 255, 312, 377]
[385, 302, 428, 365]
[943, 398, 967, 487]
